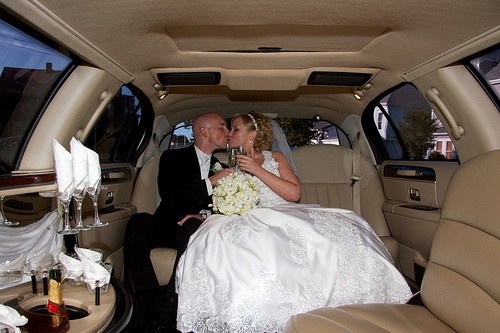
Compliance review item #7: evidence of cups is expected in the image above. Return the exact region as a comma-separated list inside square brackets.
[0, 220, 19, 227]
[234, 146, 245, 156]
[68, 252, 86, 287]
[228, 148, 236, 167]
[86, 265, 113, 295]
[89, 247, 104, 265]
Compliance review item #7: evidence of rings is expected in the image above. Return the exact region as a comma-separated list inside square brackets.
[244, 163, 245, 166]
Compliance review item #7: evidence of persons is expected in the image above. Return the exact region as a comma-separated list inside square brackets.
[123, 112, 235, 333]
[175, 112, 412, 333]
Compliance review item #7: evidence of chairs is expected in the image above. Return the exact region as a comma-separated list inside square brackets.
[124, 149, 230, 283]
[287, 149, 500, 333]
[290, 144, 399, 258]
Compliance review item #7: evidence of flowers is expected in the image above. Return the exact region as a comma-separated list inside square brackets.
[214, 172, 260, 215]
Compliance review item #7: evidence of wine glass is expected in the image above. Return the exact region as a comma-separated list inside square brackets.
[53, 173, 108, 235]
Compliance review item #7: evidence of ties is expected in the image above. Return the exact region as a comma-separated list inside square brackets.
[200, 156, 209, 180]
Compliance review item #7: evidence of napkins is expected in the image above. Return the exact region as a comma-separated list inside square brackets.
[57, 246, 115, 294]
[52, 137, 103, 207]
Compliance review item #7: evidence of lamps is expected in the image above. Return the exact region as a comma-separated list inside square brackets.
[154, 84, 167, 101]
[355, 83, 372, 101]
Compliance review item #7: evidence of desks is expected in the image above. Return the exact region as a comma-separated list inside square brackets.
[0, 263, 136, 333]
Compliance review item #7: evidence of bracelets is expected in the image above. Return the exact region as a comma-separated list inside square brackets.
[201, 214, 206, 221]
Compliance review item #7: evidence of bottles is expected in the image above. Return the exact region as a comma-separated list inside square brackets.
[47, 269, 70, 333]
